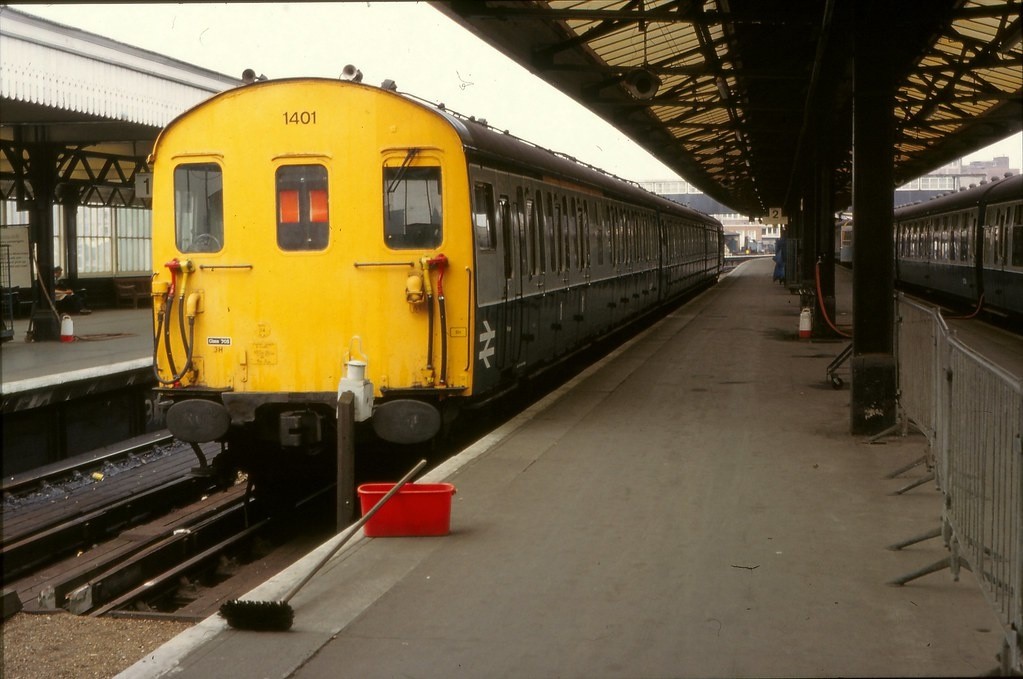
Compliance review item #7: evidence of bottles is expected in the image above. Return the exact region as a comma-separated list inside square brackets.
[60, 315, 74, 343]
[799, 308, 811, 339]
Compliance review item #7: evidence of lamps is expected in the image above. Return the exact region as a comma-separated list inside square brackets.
[716, 80, 729, 100]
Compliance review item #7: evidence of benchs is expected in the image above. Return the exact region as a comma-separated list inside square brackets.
[113, 277, 153, 310]
[0, 286, 41, 317]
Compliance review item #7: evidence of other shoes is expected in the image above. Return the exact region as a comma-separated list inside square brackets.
[80, 309, 92, 314]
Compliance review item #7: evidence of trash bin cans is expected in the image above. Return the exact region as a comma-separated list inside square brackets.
[116, 284, 137, 310]
[31, 307, 61, 341]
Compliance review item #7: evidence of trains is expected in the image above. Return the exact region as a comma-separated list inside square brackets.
[151, 64, 725, 526]
[836, 172, 1023, 333]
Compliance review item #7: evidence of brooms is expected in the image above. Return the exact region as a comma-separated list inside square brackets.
[217, 459, 427, 631]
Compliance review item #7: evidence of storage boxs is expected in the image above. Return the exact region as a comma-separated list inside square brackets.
[357, 482, 458, 537]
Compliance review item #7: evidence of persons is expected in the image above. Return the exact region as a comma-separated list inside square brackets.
[55, 266, 92, 314]
